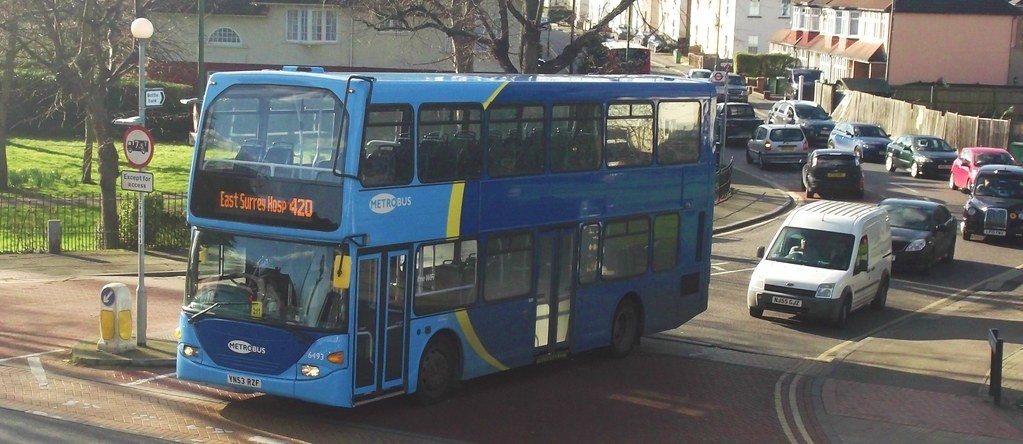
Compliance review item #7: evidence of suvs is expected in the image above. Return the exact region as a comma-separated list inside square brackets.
[764, 101, 836, 143]
[541, 16, 551, 31]
[709, 71, 749, 104]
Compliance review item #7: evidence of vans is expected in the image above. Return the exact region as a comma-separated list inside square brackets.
[784, 65, 826, 103]
[745, 198, 894, 329]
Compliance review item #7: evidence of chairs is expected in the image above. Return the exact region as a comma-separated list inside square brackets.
[435, 249, 514, 287]
[374, 127, 633, 180]
[262, 141, 294, 164]
[235, 139, 265, 162]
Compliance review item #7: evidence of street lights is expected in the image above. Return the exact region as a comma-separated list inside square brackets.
[112, 17, 154, 348]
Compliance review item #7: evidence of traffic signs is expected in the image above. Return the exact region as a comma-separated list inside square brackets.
[145, 91, 166, 106]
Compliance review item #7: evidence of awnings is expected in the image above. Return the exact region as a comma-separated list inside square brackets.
[771, 28, 884, 63]
[791, 0, 893, 12]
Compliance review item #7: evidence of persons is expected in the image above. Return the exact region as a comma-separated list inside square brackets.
[256, 276, 279, 318]
[789, 236, 812, 256]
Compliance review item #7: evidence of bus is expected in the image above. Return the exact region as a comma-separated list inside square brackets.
[177, 63, 718, 408]
[600, 42, 651, 74]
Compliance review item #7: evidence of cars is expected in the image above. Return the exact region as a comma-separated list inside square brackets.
[745, 124, 813, 170]
[611, 25, 674, 54]
[885, 135, 958, 180]
[876, 198, 957, 275]
[827, 122, 894, 164]
[949, 146, 1019, 193]
[961, 164, 1023, 240]
[688, 69, 712, 81]
[800, 148, 865, 198]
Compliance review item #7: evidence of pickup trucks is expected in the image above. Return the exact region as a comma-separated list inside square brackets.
[715, 101, 765, 143]
